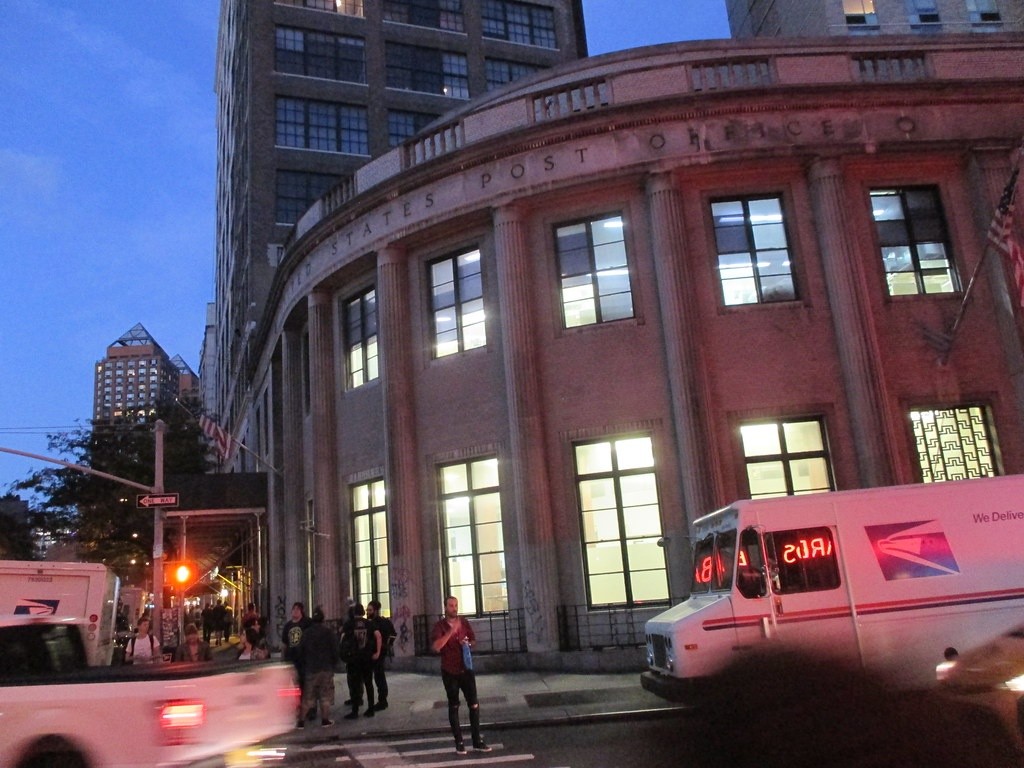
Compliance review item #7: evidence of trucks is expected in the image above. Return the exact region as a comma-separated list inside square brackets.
[642, 475, 1024, 701]
[0, 560, 146, 668]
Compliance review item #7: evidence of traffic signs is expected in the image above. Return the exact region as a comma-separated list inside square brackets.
[136, 493, 180, 508]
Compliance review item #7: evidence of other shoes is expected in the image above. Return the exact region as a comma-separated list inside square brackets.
[374, 702, 388, 711]
[344, 712, 358, 720]
[296, 720, 305, 729]
[344, 699, 364, 706]
[455, 741, 467, 754]
[473, 742, 492, 752]
[363, 708, 375, 717]
[321, 720, 335, 728]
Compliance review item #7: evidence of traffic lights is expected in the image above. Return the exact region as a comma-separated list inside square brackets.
[164, 561, 198, 586]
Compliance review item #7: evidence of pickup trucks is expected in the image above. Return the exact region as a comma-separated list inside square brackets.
[0, 615, 302, 768]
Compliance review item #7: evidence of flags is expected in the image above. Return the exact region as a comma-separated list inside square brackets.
[179, 403, 236, 461]
[983, 135, 1024, 315]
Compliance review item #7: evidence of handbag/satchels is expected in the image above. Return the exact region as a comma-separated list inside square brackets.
[464, 644, 474, 671]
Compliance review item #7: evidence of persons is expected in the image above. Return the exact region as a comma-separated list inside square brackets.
[111, 598, 235, 648]
[430, 594, 493, 754]
[343, 601, 365, 706]
[295, 609, 336, 730]
[241, 602, 261, 631]
[281, 601, 318, 720]
[338, 603, 381, 719]
[127, 618, 161, 665]
[257, 617, 272, 659]
[366, 600, 390, 711]
[235, 627, 266, 660]
[243, 618, 266, 647]
[174, 622, 213, 664]
